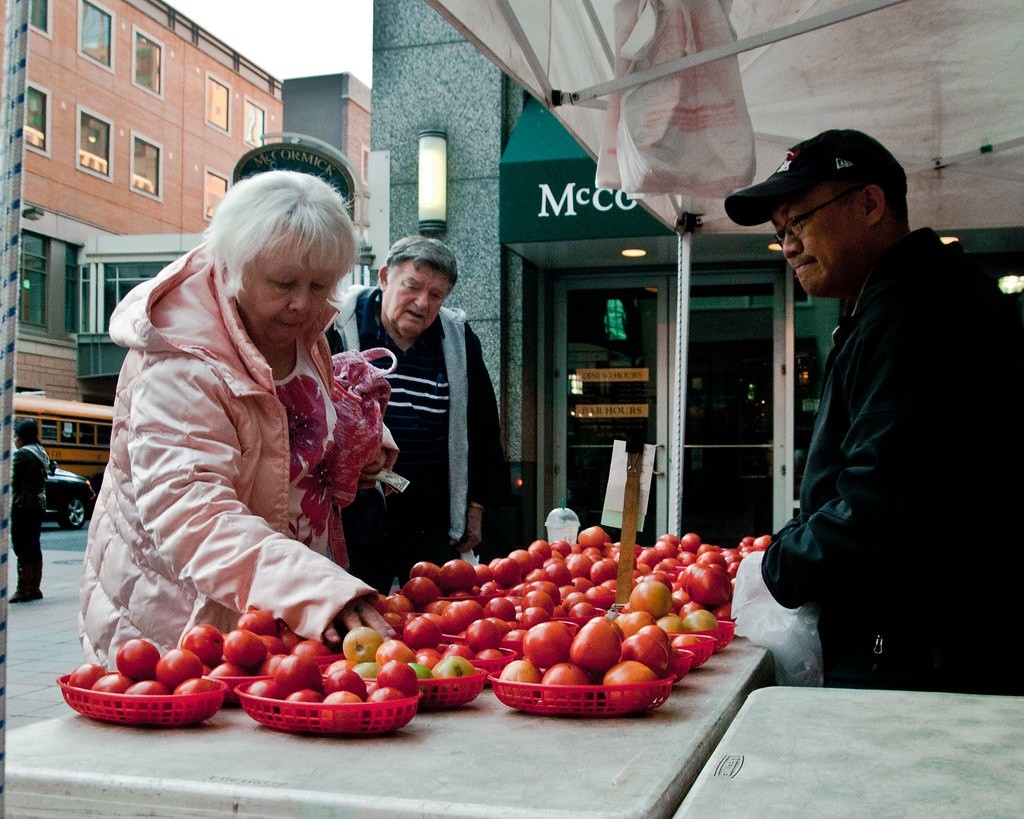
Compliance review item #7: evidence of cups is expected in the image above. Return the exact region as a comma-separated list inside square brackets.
[545, 507, 581, 545]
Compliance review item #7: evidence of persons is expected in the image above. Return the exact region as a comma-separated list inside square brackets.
[322, 231, 501, 594]
[723, 130, 1024, 697]
[7, 419, 50, 604]
[68, 170, 400, 676]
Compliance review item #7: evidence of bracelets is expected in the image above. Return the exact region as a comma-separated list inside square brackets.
[471, 501, 483, 510]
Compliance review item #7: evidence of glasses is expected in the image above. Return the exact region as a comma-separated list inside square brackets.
[773, 184, 866, 248]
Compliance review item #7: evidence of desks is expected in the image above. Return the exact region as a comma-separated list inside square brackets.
[5, 635, 776, 819]
[669, 685, 1024, 819]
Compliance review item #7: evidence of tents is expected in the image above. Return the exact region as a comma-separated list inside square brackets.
[422, 0, 1024, 540]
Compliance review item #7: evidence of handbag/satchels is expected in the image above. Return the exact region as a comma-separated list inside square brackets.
[318, 347, 398, 507]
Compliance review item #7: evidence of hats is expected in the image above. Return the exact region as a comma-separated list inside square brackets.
[724, 129, 905, 226]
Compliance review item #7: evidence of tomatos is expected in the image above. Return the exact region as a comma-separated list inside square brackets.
[68, 525, 773, 731]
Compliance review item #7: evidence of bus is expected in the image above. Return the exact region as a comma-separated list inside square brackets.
[13, 392, 114, 494]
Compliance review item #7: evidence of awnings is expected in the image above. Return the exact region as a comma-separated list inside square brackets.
[496, 92, 791, 267]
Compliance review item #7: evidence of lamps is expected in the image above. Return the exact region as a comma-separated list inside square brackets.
[23, 206, 45, 220]
[418, 130, 447, 235]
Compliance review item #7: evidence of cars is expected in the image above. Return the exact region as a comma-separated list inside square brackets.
[44, 458, 97, 530]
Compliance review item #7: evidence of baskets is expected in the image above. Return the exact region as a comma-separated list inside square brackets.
[56, 537, 738, 735]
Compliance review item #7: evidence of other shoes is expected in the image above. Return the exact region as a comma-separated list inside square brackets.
[8, 590, 43, 603]
